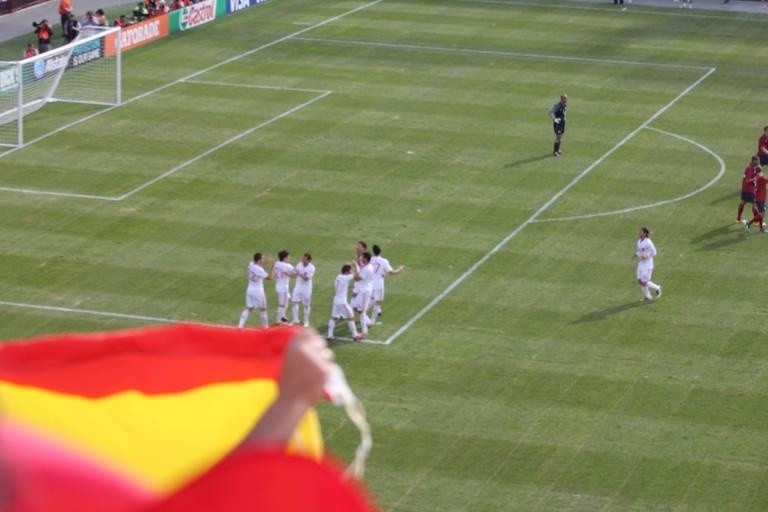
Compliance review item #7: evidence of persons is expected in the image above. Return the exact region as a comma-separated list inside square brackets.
[274, 252, 297, 325]
[757, 126, 768, 166]
[240, 327, 335, 450]
[22, 0, 201, 60]
[744, 166, 768, 232]
[237, 253, 275, 329]
[289, 254, 315, 326]
[549, 95, 568, 156]
[633, 228, 661, 301]
[736, 156, 766, 225]
[326, 241, 404, 341]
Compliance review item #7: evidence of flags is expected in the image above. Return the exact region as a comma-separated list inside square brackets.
[0, 323, 324, 493]
